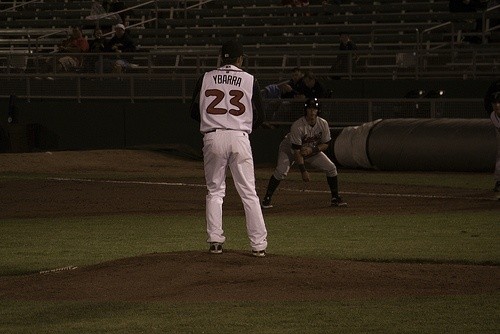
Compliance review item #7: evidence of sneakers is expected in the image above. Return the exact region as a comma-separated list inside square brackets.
[209, 242, 223, 253]
[252, 250, 265, 256]
[331, 197, 348, 207]
[261, 200, 273, 208]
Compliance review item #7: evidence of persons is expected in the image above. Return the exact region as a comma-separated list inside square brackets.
[59, 0, 137, 81]
[448, 0, 488, 13]
[337, 32, 364, 65]
[488, 93, 500, 192]
[191, 42, 291, 257]
[286, 0, 344, 34]
[280, 68, 331, 99]
[261, 98, 347, 208]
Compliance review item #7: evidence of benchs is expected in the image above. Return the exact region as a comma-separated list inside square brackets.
[0, 0, 500, 77]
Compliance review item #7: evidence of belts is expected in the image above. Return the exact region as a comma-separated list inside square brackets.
[206, 129, 216, 134]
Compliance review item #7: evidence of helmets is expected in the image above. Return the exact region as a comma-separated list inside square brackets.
[304, 97, 320, 116]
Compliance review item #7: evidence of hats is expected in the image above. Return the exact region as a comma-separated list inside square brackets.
[115, 24, 125, 30]
[490, 92, 500, 103]
[220, 37, 245, 57]
